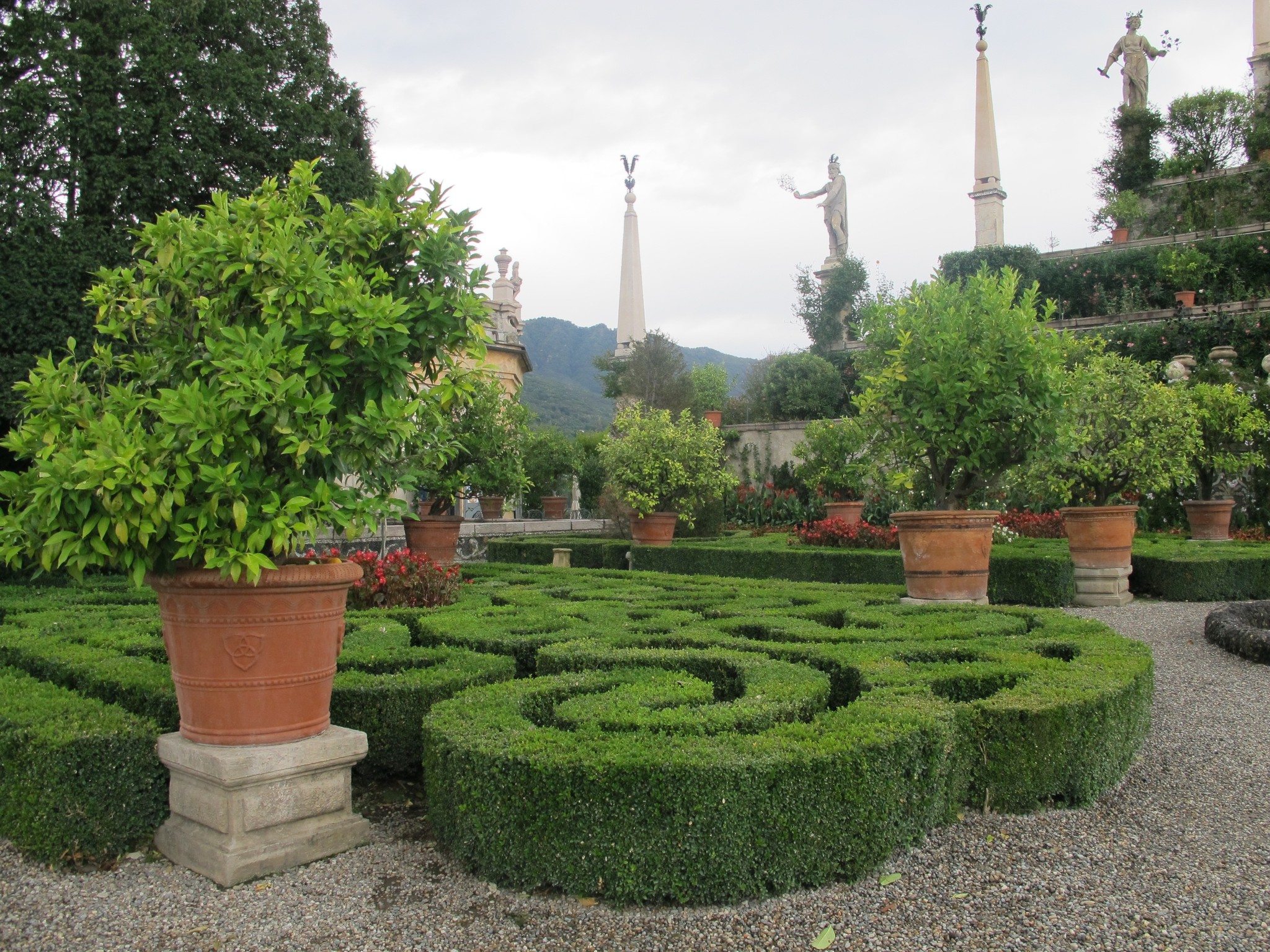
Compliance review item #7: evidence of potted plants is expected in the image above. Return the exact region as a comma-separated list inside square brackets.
[0, 93, 1270, 748]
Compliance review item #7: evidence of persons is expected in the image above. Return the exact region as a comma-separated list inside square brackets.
[794, 154, 849, 259]
[1101, 10, 1167, 112]
